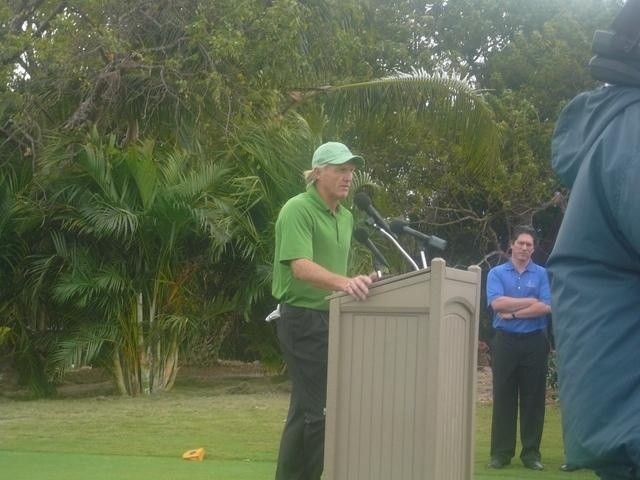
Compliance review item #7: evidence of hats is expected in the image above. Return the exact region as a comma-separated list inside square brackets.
[312, 141, 365, 170]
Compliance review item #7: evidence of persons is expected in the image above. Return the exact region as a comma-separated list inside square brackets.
[268, 137, 380, 480]
[477, 228, 559, 469]
[552, 1, 638, 480]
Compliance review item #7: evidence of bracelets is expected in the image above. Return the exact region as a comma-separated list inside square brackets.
[512, 311, 516, 320]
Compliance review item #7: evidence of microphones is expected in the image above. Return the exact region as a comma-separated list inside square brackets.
[390, 219, 447, 251]
[353, 193, 398, 240]
[353, 229, 392, 268]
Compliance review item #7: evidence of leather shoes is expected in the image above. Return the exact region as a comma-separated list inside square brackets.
[488, 456, 512, 469]
[523, 457, 545, 470]
[560, 462, 584, 471]
